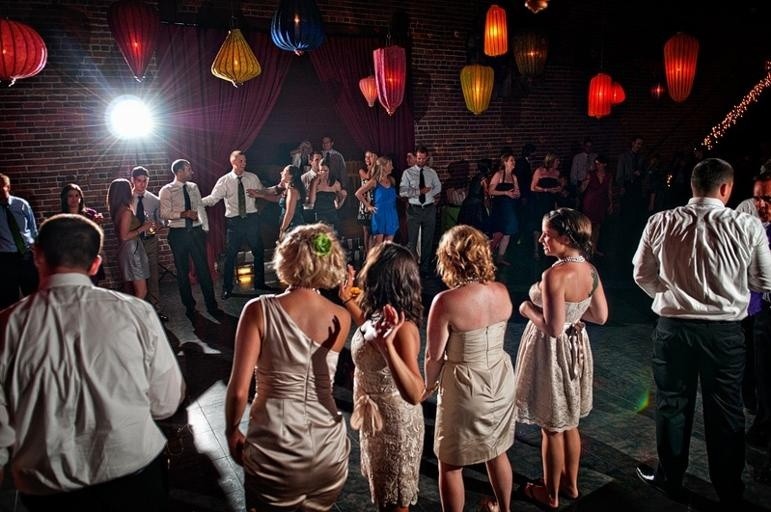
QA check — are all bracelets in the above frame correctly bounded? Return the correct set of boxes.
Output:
[135,229,141,236]
[343,296,354,304]
[425,381,439,392]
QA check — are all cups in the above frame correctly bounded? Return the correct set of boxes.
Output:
[191,207,199,221]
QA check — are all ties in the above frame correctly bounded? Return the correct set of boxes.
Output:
[134,196,146,240]
[183,184,193,230]
[326,152,330,167]
[419,168,427,205]
[237,175,247,219]
[4,206,27,259]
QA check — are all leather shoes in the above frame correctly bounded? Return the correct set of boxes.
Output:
[185,296,196,316]
[637,466,683,501]
[158,312,170,321]
[221,289,233,300]
[253,280,275,292]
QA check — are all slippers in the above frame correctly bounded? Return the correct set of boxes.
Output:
[538,475,583,502]
[519,481,560,511]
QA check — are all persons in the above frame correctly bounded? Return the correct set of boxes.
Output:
[0,214,188,511]
[0,171,38,310]
[419,223,520,512]
[158,156,219,317]
[288,136,705,281]
[631,156,771,510]
[511,205,610,512]
[60,183,107,288]
[107,175,150,299]
[127,165,168,323]
[335,238,425,512]
[245,164,306,242]
[200,150,283,300]
[224,221,356,512]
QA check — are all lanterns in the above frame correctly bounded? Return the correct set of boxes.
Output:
[1,15,50,89]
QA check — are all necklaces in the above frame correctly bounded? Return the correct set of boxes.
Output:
[552,255,587,267]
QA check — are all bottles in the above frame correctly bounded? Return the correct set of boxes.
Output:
[145,212,156,235]
